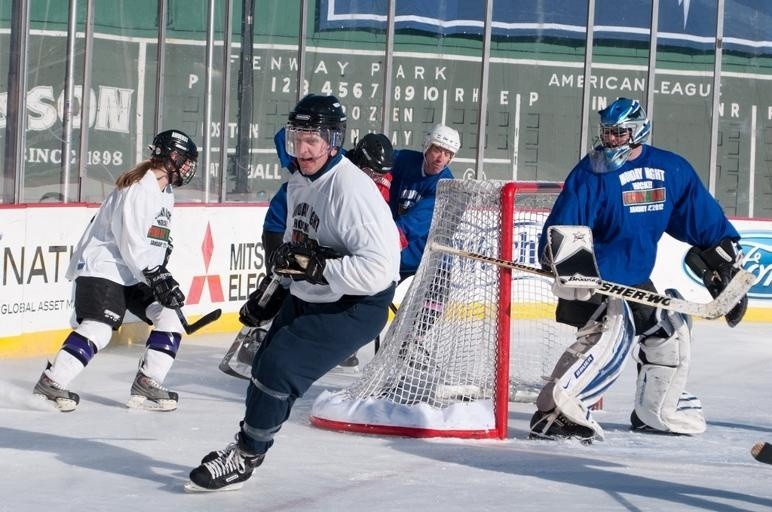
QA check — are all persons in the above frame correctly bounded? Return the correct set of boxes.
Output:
[32,129,199,412]
[184,94,401,492]
[527,97,748,442]
[392,126,469,370]
[237,128,407,367]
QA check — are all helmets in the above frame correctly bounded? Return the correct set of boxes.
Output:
[422,124,462,169]
[355,133,394,174]
[150,129,199,188]
[284,93,347,178]
[589,96,651,174]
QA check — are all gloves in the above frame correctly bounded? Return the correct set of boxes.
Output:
[238,275,292,327]
[265,237,343,285]
[542,224,602,302]
[143,265,185,309]
[682,236,748,327]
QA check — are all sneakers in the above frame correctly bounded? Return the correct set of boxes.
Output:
[527,408,596,447]
[124,369,180,403]
[29,370,81,405]
[188,434,269,490]
[629,404,708,438]
